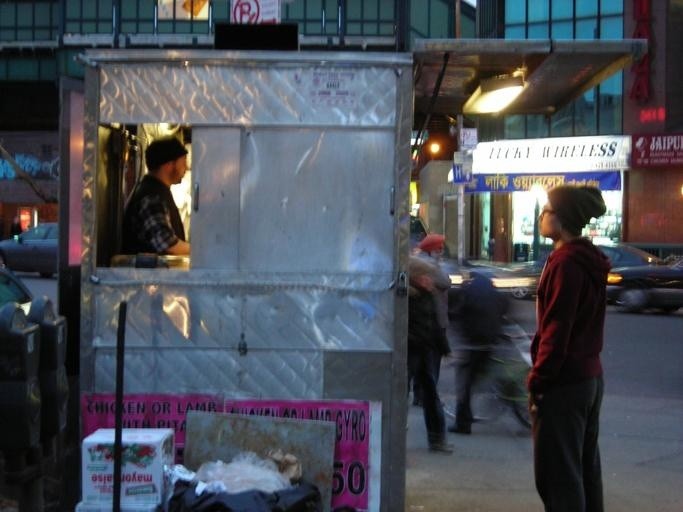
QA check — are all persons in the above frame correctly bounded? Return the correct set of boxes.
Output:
[524,182,612,511]
[10,215,21,239]
[404,232,452,409]
[445,269,517,436]
[121,135,191,255]
[405,256,454,454]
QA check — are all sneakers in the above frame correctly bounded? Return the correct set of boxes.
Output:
[448,424,472,435]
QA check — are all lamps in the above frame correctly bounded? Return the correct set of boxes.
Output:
[462,74,530,116]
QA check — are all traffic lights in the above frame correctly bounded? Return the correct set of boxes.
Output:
[423,133,456,160]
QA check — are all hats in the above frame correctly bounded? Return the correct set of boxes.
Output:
[419,234,444,254]
[549,186,605,228]
[145,138,189,172]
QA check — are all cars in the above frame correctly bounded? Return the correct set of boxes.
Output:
[1,266,42,408]
[1,220,61,279]
[407,210,683,326]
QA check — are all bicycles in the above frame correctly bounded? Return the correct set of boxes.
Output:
[434,321,533,428]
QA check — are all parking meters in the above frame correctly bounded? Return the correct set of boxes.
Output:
[27,295,70,437]
[1,302,43,452]
